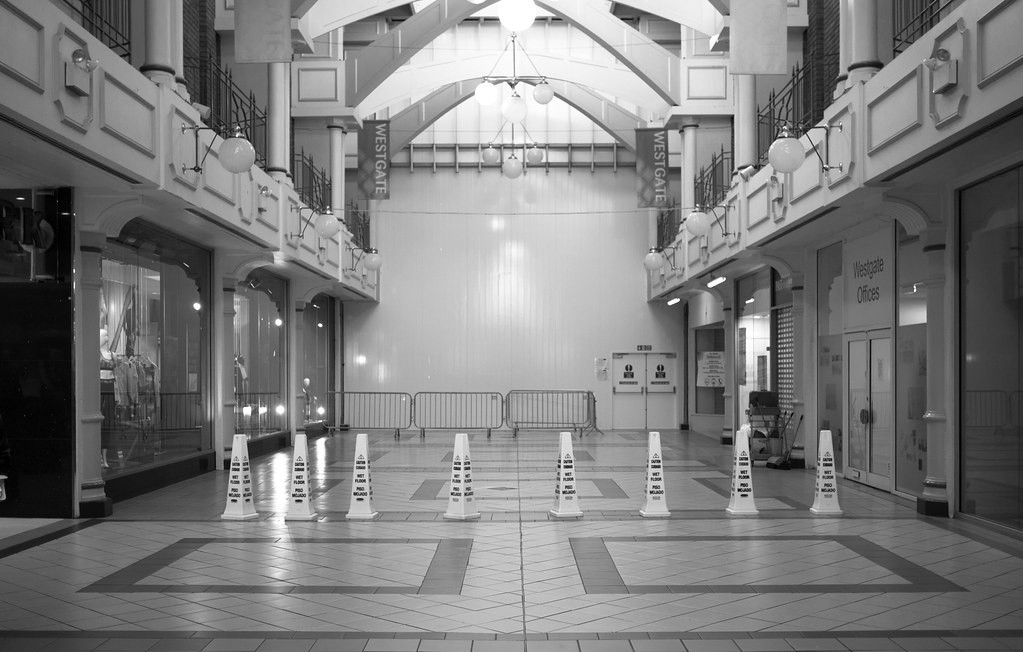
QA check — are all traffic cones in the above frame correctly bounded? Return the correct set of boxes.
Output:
[640,431,672,518]
[285,434,319,521]
[548,432,583,518]
[726,431,759,514]
[345,434,379,519]
[220,435,259,519]
[810,430,844,515]
[444,433,481,520]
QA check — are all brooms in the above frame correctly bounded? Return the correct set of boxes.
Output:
[756,399,804,470]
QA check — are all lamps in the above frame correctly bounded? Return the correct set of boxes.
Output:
[169,120,256,181]
[661,286,683,306]
[347,247,383,271]
[768,176,783,201]
[645,244,680,273]
[707,268,728,291]
[768,122,843,180]
[290,205,339,240]
[471,2,553,176]
[63,50,98,99]
[687,203,736,237]
[259,185,271,210]
[925,45,959,94]
